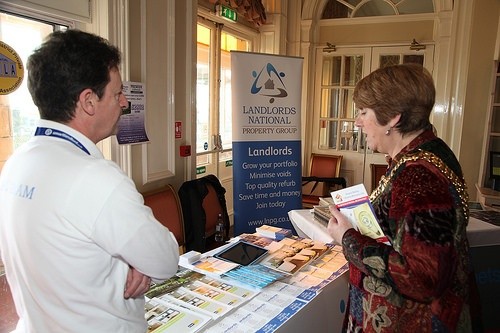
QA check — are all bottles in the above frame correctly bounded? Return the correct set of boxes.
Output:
[214,213,224,241]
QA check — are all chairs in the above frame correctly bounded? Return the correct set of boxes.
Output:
[141,183,186,254]
[179,175,230,252]
[369,163,387,192]
[302,153,343,209]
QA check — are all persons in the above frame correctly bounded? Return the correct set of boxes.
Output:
[327,62,477,333]
[0,28,179,333]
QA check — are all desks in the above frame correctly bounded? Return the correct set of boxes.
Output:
[144,235,354,333]
[466,208,500,248]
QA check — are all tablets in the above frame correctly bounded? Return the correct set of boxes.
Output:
[215,241,269,266]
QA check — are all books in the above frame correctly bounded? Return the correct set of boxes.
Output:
[143,224,349,333]
[313,198,335,227]
